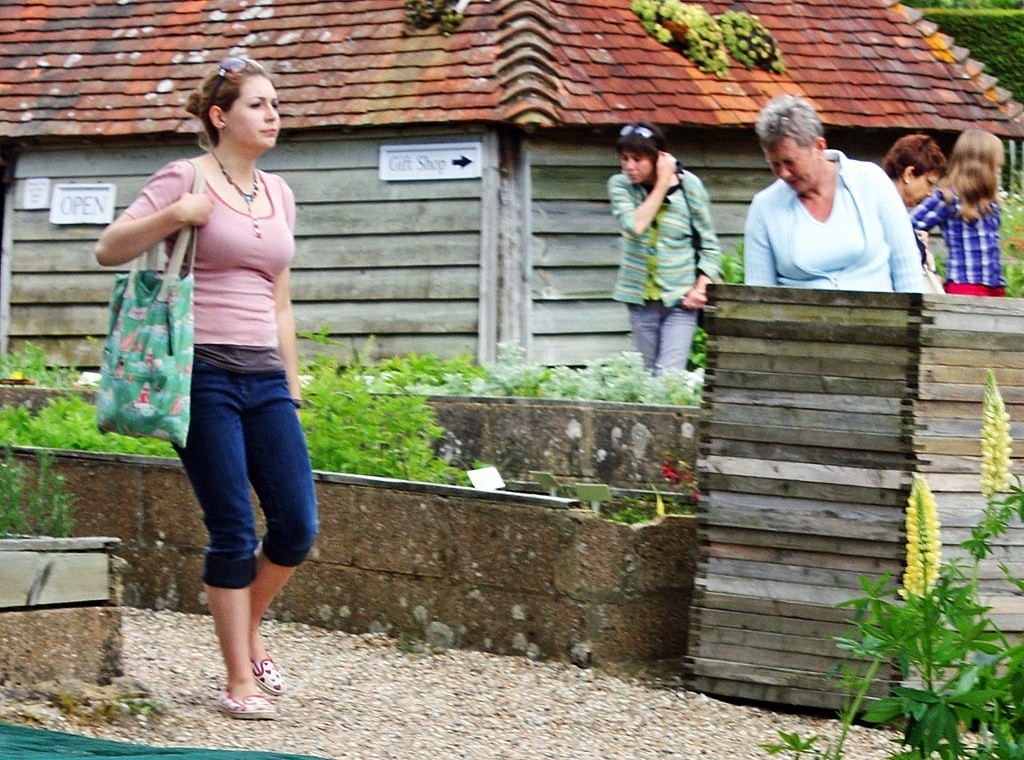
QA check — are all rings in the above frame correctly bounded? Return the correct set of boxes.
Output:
[691,303,695,306]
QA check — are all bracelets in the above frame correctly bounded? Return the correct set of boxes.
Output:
[693,284,706,295]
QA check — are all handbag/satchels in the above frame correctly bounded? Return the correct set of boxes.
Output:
[97,162,206,449]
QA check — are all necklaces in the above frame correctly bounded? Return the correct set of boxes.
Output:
[211,151,262,240]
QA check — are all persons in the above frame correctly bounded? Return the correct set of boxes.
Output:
[885,134,946,267]
[93,58,319,718]
[606,122,721,377]
[743,94,924,292]
[909,129,1008,298]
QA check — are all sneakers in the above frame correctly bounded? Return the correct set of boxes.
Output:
[216,687,276,720]
[251,651,287,696]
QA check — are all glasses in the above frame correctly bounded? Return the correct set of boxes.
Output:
[618,124,658,152]
[211,56,265,107]
